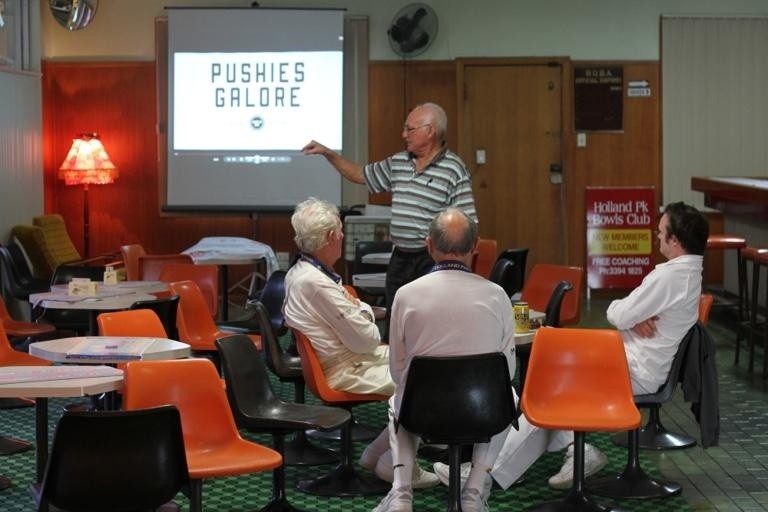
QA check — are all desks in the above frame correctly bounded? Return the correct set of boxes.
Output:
[514,327,553,395]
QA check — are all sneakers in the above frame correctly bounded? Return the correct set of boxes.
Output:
[359,444,609,511]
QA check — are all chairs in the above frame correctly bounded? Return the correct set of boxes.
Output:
[473,240,496,278]
[521,264,582,325]
[521,327,641,508]
[397,352,519,512]
[610,294,712,449]
[488,247,529,296]
[489,258,513,288]
[585,424,683,500]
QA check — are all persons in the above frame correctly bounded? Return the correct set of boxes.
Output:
[433,202,710,492]
[281,197,441,489]
[370,206,520,512]
[301,103,479,344]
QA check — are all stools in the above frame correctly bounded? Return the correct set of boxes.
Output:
[702,234,750,346]
[740,244,768,377]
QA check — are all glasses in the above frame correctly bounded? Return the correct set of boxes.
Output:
[400,124,430,133]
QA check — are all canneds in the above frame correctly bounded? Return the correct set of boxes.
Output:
[514,302,529,334]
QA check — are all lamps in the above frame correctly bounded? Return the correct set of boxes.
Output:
[59,134,115,260]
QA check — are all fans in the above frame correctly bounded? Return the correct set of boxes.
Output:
[384,3,439,58]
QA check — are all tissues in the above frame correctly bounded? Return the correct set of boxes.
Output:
[103,267,118,285]
[68,277,100,296]
